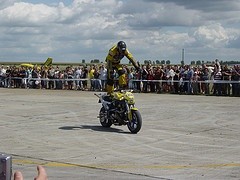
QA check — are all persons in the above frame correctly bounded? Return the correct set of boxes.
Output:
[118,63,240,97]
[105,40,140,92]
[0,64,119,92]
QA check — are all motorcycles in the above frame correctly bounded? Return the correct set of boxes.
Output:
[94,88,142,134]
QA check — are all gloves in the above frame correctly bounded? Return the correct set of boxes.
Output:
[110,71,116,81]
[136,67,143,75]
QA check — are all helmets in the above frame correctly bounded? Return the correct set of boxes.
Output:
[118,41,126,50]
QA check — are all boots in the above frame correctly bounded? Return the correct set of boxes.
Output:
[119,74,126,85]
[107,84,114,98]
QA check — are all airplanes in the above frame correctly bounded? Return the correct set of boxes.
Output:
[20,57,53,71]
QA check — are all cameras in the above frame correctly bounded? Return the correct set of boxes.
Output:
[0,154,12,180]
